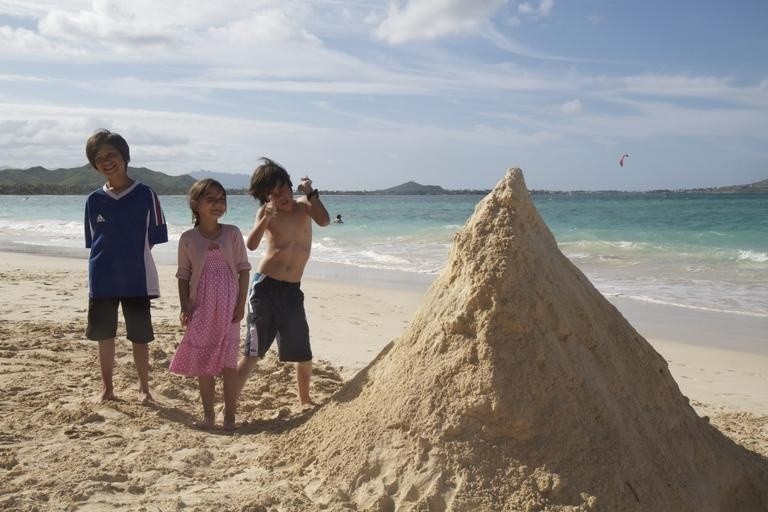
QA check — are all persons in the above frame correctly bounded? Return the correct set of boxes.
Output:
[85,129,167,406]
[235,157,330,406]
[169,178,251,431]
[334,214,343,223]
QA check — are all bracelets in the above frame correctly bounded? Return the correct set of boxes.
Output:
[308,188,319,201]
